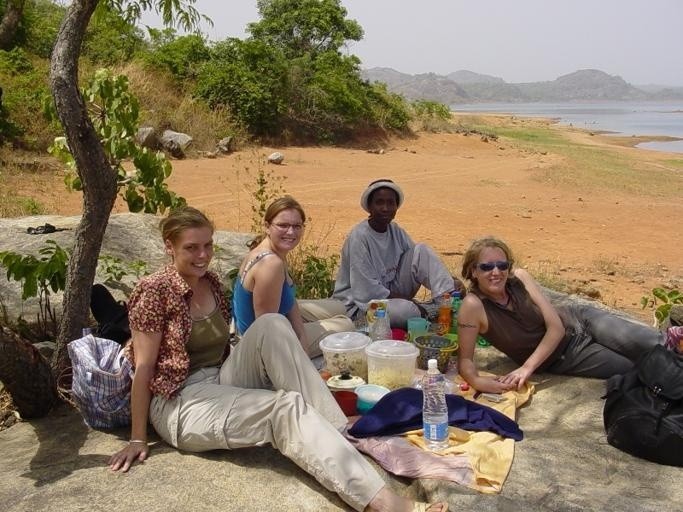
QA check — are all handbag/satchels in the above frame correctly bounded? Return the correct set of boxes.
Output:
[66,334,134,429]
[599,343,683,467]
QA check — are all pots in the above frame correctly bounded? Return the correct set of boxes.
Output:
[326,370,366,391]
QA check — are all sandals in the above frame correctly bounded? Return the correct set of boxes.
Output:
[407,500,449,512]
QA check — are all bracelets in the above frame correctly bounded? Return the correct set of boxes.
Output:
[129,439,147,443]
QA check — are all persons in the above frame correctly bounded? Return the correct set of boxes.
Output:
[108,206,451,512]
[330,178,466,331]
[457,236,673,393]
[232,194,354,360]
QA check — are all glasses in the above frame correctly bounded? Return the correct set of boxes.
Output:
[271,222,303,231]
[474,260,510,271]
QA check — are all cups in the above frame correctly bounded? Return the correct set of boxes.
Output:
[335,391,357,416]
[407,317,431,341]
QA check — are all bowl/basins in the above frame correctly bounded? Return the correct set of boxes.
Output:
[354,384,391,416]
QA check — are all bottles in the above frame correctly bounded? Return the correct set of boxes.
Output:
[422,358,449,452]
[414,373,470,394]
[437,291,451,335]
[452,291,461,334]
[366,302,392,340]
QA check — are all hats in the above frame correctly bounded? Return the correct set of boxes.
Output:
[359,178,404,214]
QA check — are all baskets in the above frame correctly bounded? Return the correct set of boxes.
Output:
[413,335,459,374]
